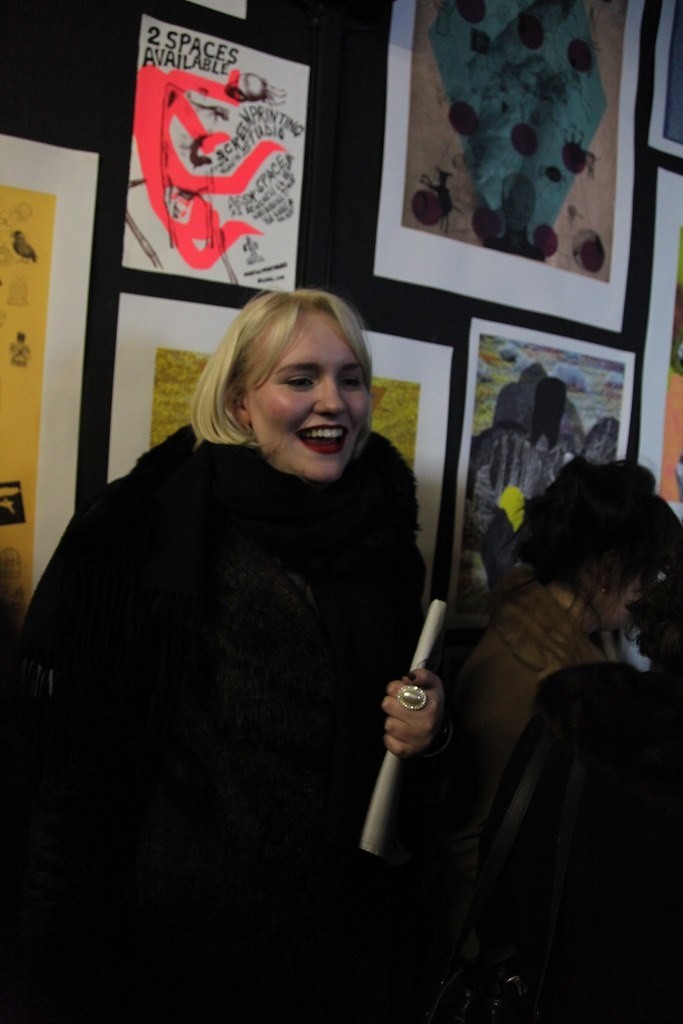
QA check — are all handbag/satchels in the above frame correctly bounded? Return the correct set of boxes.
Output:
[425,718,588,1023]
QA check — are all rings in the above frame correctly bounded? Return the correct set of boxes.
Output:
[397,684,429,710]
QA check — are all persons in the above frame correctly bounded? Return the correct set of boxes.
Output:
[440,451,683,1024]
[2,285,482,1024]
